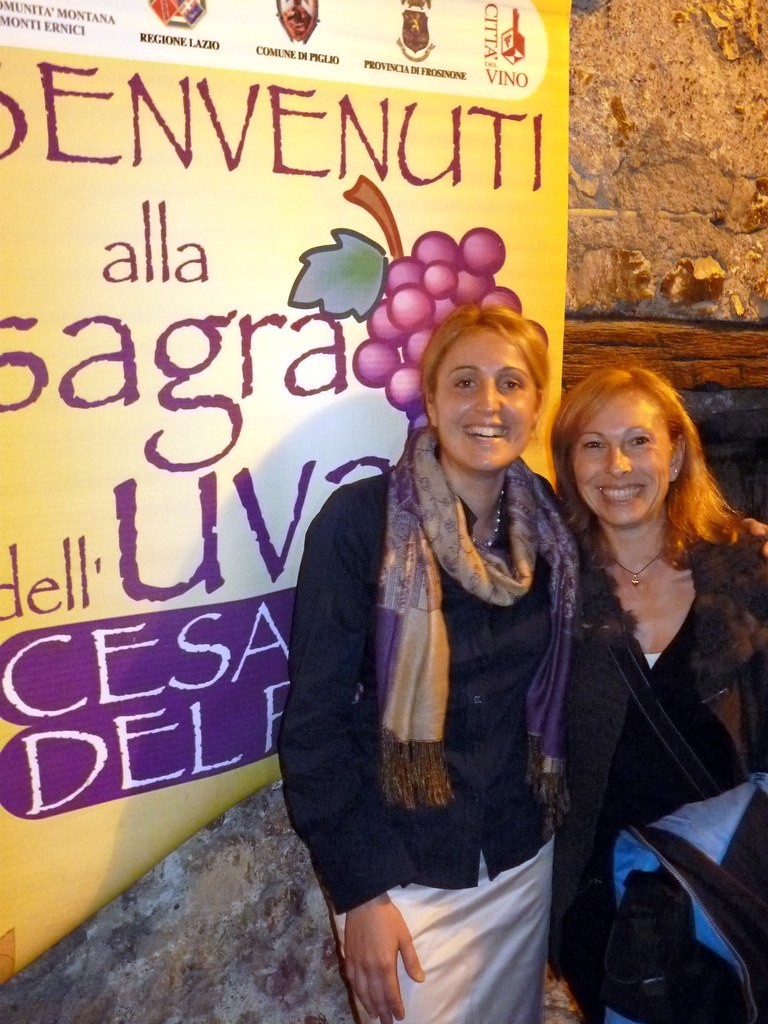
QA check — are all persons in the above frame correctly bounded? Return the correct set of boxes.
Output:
[277,303,581,1024]
[551,366,768,1024]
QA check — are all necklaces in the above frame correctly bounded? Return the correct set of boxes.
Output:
[610,552,662,586]
[471,491,504,547]
